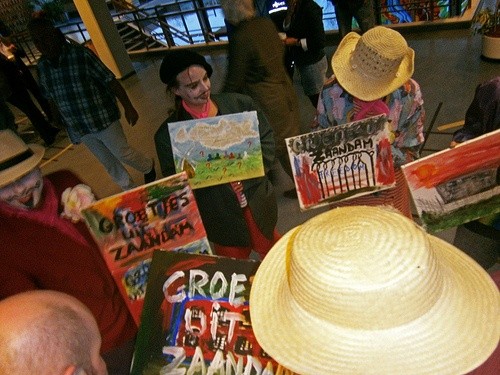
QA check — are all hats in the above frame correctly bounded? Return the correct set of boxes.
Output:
[249,205,500,375]
[159,48,213,84]
[0,129,45,188]
[331,26,415,101]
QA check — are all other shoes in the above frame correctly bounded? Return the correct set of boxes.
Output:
[144,158,156,184]
[311,112,319,128]
[283,188,298,199]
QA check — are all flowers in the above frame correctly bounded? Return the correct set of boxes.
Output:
[470,0,500,40]
[58,183,96,223]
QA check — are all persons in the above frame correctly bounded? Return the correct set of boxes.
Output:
[281,0,328,110]
[31,18,157,191]
[0,128,138,375]
[221,0,299,200]
[0,27,64,145]
[310,24,425,219]
[0,287,111,375]
[153,47,283,259]
[266,0,384,87]
[448,74,500,277]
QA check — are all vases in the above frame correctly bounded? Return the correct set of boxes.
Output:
[481,33,500,59]
